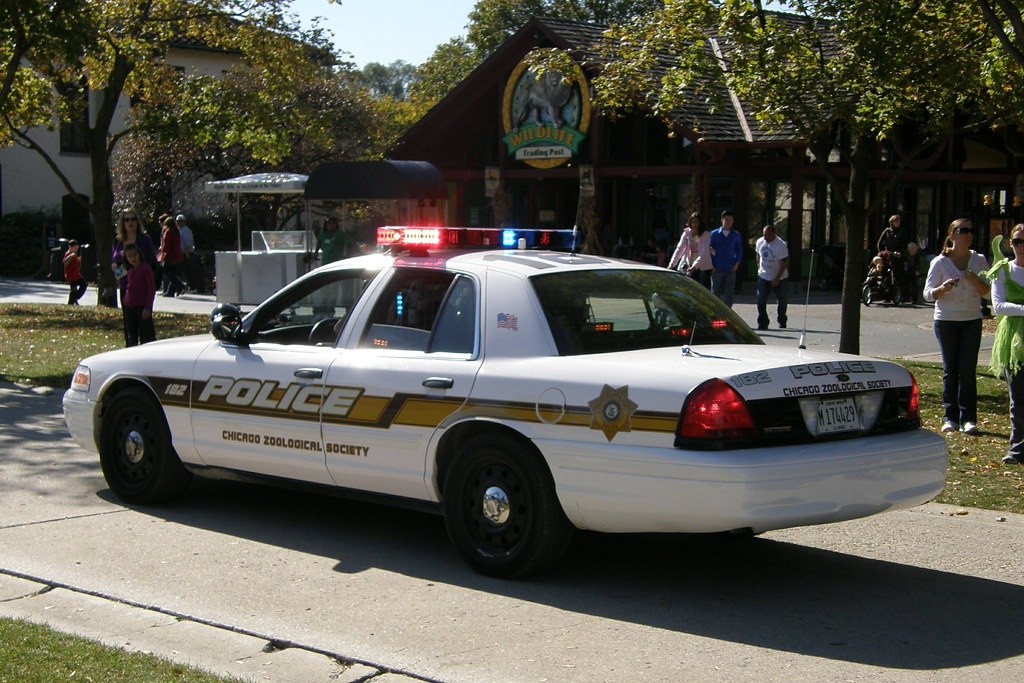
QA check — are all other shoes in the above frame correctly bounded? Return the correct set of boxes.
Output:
[163,292,174,297]
[778,324,787,328]
[757,323,768,330]
[176,282,187,296]
[1002,454,1018,464]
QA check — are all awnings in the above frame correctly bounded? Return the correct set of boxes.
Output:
[303,158,447,251]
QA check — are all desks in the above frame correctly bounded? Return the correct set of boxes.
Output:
[213,249,320,306]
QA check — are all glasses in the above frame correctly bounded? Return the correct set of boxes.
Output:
[1011,237,1024,247]
[954,227,975,235]
[123,216,138,222]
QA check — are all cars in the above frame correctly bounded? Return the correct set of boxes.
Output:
[60,223,950,582]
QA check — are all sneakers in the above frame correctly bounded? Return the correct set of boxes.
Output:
[941,420,960,433]
[958,421,978,433]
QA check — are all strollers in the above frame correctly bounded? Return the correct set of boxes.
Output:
[861,250,904,306]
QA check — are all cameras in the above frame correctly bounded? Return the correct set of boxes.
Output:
[949,277,960,287]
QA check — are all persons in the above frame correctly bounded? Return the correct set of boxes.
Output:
[63,239,88,305]
[666,211,716,293]
[1011,195,1021,206]
[754,224,788,329]
[990,222,1024,464]
[877,214,908,274]
[156,214,187,297]
[898,242,922,306]
[868,255,900,305]
[709,208,743,310]
[983,194,992,206]
[922,218,993,432]
[111,208,160,305]
[122,243,157,347]
[175,213,199,294]
[314,215,356,318]
[641,235,661,267]
